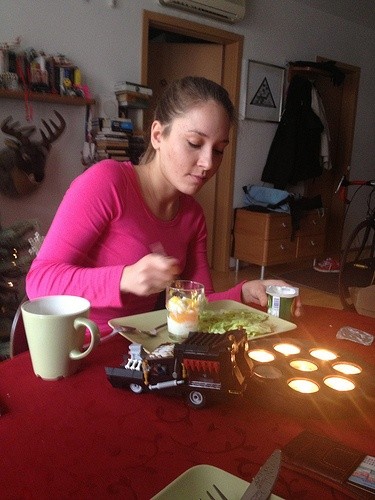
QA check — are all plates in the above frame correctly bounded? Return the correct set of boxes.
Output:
[109,299,296,356]
[149,464,285,500]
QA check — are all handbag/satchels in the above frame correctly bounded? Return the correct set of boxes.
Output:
[241,185,293,211]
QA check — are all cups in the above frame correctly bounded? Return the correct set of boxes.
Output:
[20,295,99,381]
[264,286,298,323]
[165,280,204,342]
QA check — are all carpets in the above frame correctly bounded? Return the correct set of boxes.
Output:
[275,257,375,299]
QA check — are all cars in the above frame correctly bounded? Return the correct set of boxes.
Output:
[104,324,256,410]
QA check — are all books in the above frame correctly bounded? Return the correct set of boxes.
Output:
[92,118,144,161]
[115,81,153,107]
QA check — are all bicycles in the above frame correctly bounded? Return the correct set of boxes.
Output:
[333,175,375,314]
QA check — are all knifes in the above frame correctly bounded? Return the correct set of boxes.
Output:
[239,449,282,500]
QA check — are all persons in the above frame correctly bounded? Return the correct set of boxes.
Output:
[27,77,301,349]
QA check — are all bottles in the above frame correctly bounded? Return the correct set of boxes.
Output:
[0,37,84,97]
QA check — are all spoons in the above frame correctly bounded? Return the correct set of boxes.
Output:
[139,321,167,337]
[83,325,136,350]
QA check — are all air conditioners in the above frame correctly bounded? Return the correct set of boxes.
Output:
[156,0,249,23]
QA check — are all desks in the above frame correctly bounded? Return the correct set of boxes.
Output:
[0,304,374,500]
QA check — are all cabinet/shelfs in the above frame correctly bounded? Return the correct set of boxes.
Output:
[234,209,330,279]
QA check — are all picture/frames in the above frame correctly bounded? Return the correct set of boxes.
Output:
[244,60,285,123]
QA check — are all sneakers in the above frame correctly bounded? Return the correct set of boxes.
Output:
[313,258,339,272]
